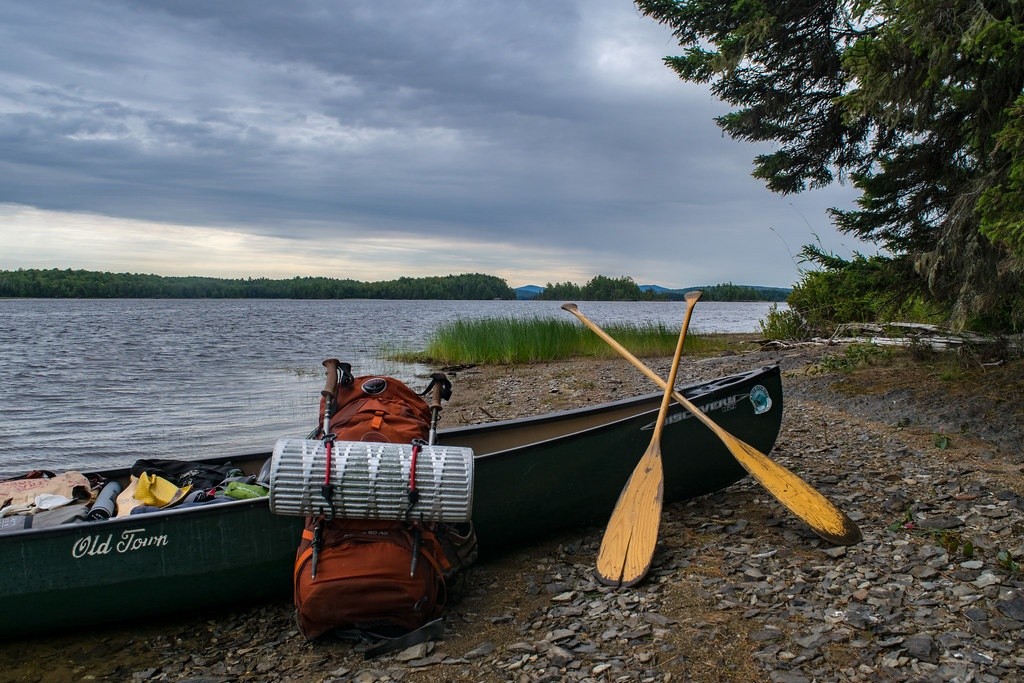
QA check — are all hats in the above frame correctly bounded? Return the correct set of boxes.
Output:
[133,470,194,510]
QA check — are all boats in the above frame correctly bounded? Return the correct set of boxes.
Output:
[0,360,788,649]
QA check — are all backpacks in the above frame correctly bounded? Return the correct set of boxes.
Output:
[130,456,245,491]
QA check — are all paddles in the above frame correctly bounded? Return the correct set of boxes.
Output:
[560,291,864,589]
